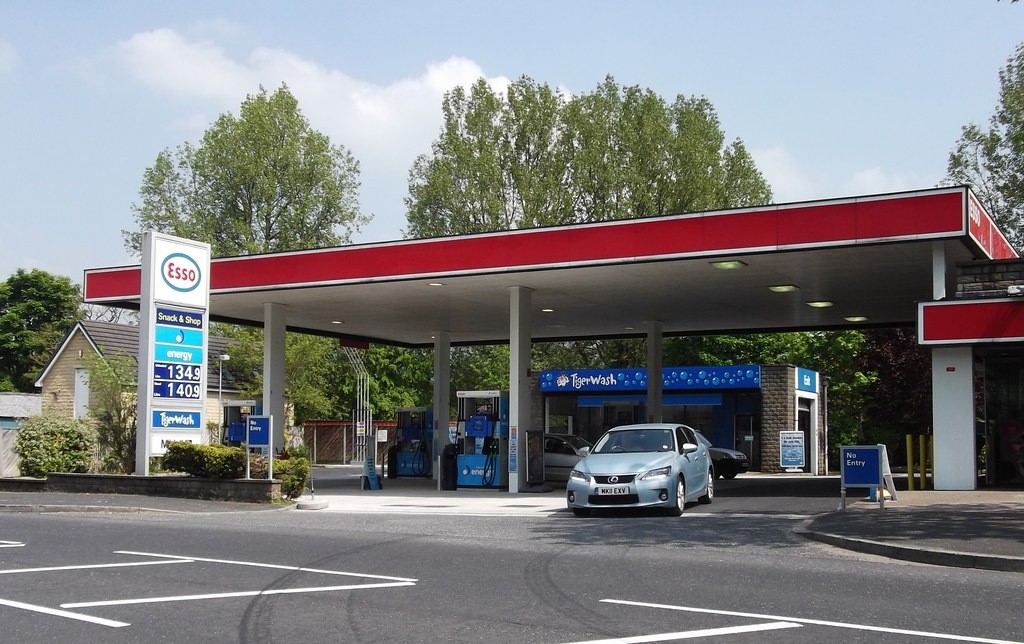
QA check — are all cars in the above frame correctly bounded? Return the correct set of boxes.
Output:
[565,424,716,516]
[694,429,747,480]
[540,433,598,481]
